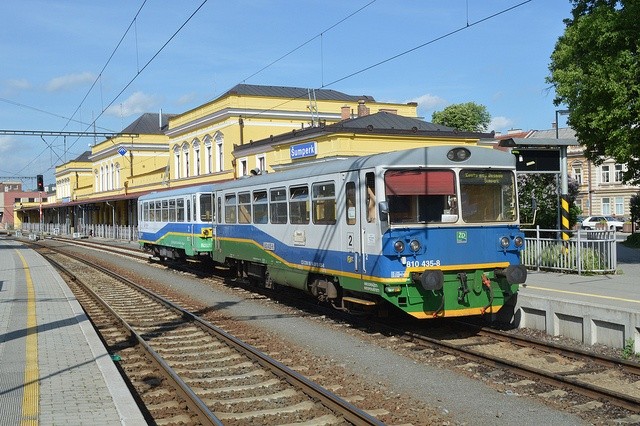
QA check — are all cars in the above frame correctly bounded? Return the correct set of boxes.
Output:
[581,216,624,232]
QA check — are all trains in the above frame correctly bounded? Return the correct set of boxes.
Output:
[137,145,527,328]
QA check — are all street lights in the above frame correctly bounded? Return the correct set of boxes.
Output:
[555,110,572,245]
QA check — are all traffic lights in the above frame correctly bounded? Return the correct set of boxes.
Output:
[37,175,44,191]
